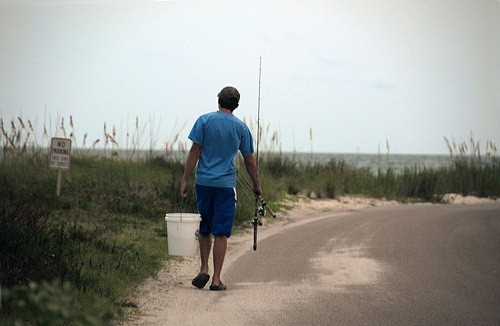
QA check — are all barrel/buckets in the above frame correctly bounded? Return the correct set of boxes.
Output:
[165,188,201,257]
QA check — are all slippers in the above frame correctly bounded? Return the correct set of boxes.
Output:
[192,272,210,289]
[210,280,227,290]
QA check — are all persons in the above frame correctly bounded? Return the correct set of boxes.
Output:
[180,86,262,291]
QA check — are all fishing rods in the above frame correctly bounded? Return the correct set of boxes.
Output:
[252,57,264,250]
[235,164,278,219]
